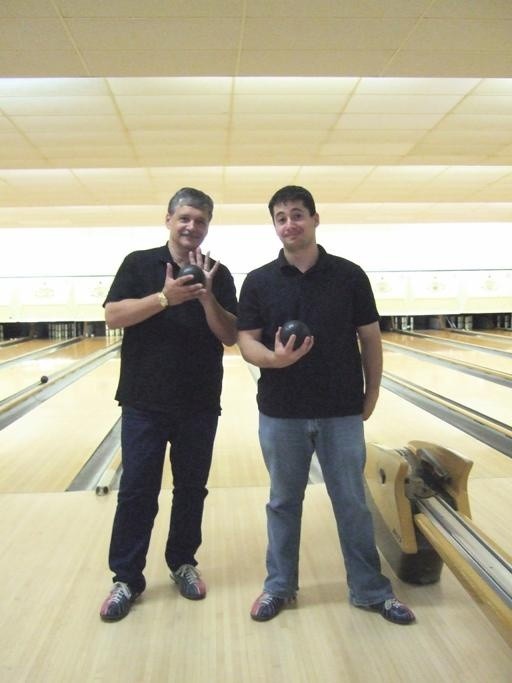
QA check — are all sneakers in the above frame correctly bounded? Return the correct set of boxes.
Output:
[352,591,415,624]
[251,587,297,622]
[169,560,207,600]
[99,577,145,622]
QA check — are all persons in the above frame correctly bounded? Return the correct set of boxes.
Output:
[234,181,417,625]
[96,186,239,622]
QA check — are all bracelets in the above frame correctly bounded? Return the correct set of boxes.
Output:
[158,289,172,310]
